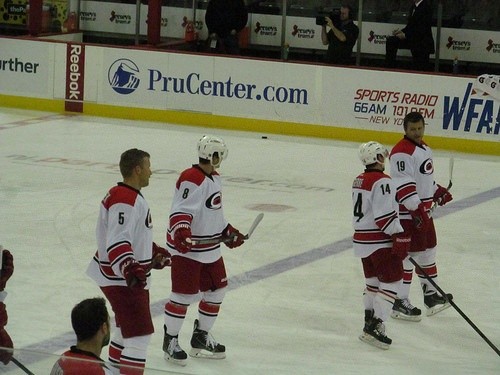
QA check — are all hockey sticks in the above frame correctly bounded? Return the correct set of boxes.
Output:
[9,356,35,375]
[408,256,500,356]
[412,157,453,227]
[128,254,162,287]
[191,213,264,246]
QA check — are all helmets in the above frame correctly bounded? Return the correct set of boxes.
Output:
[358,140,389,166]
[196,136,228,168]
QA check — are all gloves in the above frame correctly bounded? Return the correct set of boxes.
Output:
[151,242,173,270]
[0,328,14,365]
[0,249,14,292]
[120,258,147,289]
[173,224,194,253]
[0,302,9,329]
[407,203,431,233]
[433,184,453,206]
[222,224,247,249]
[391,231,411,260]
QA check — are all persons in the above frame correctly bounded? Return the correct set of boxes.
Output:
[0,245,14,365]
[320,4,359,64]
[203,0,248,55]
[351,140,410,350]
[389,112,455,321]
[162,133,245,366]
[50,296,114,375]
[386,0,435,72]
[87,148,172,375]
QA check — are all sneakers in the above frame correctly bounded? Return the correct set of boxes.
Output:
[161,323,187,367]
[188,319,227,359]
[359,310,392,350]
[391,299,422,321]
[421,282,453,318]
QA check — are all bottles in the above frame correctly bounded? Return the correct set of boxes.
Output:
[453,57,458,73]
[283,43,289,60]
